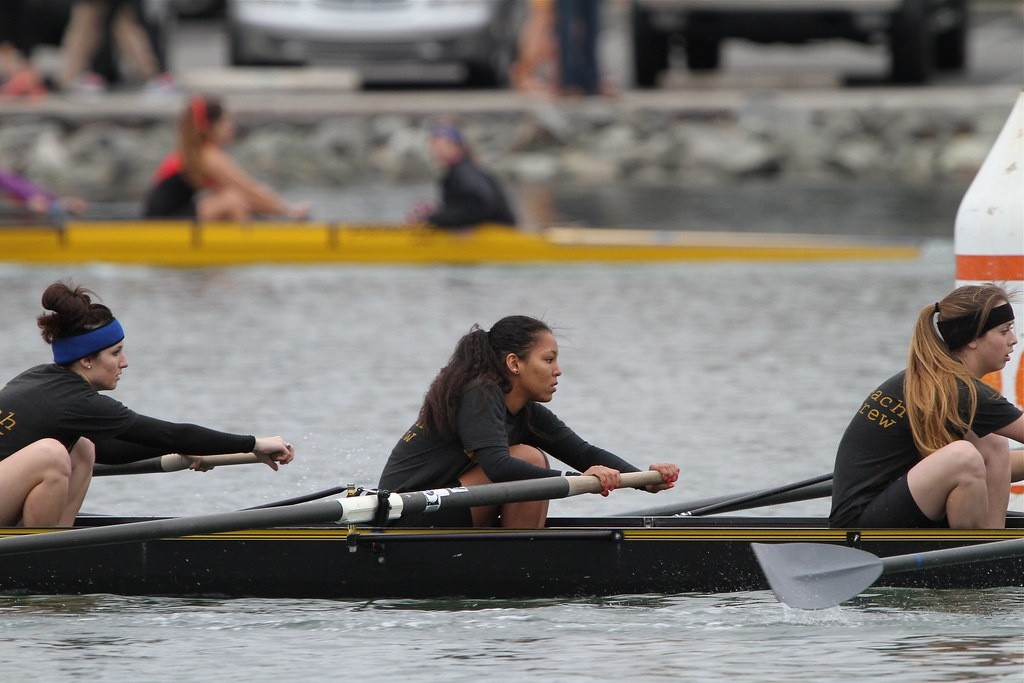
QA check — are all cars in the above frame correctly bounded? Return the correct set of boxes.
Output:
[605,1,977,89]
[215,1,532,94]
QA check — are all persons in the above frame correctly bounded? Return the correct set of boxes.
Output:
[415,120,515,233]
[140,97,311,220]
[0,0,175,98]
[0,284,294,527]
[0,169,85,214]
[828,284,1024,530]
[550,0,613,103]
[378,315,681,528]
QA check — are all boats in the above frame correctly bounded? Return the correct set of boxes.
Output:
[0,508,1024,605]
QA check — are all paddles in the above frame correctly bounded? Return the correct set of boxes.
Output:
[751,538,1024,610]
[0,470,664,556]
[93,449,283,477]
[610,478,834,518]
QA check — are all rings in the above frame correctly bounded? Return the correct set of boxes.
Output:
[286,444,291,449]
